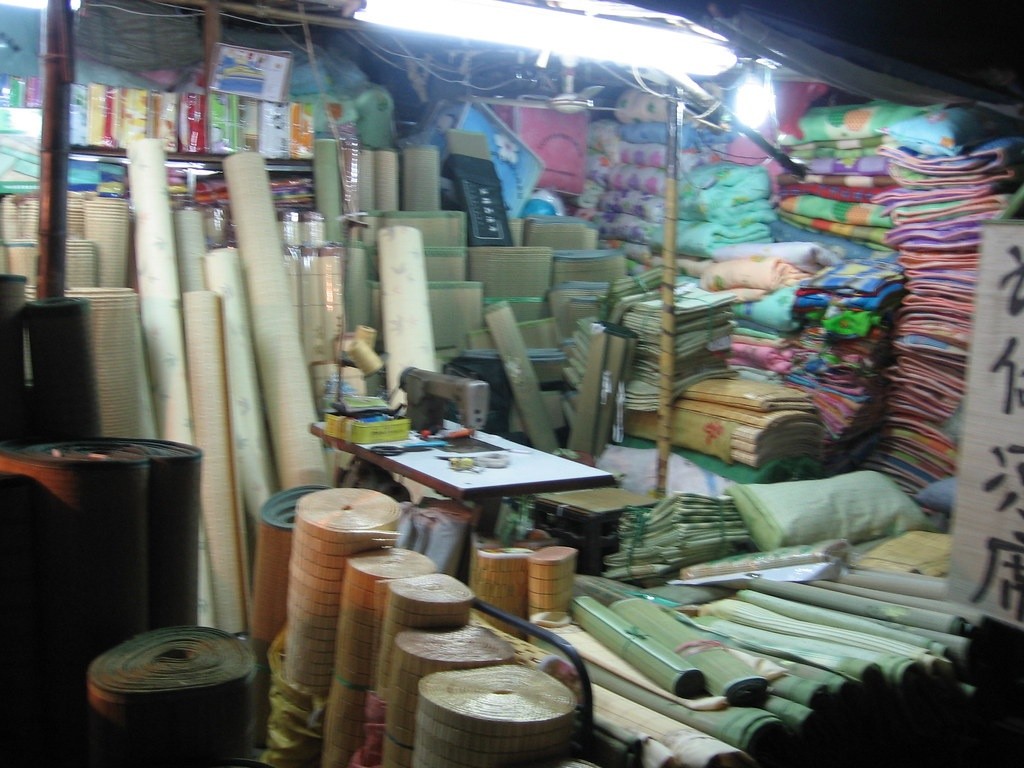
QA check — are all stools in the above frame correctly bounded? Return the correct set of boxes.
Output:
[528,486,658,580]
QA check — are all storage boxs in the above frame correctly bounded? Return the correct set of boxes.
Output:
[425,96,589,212]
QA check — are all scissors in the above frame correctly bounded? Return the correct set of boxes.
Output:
[371,444,431,457]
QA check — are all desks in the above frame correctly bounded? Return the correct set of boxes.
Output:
[309,410,615,581]
